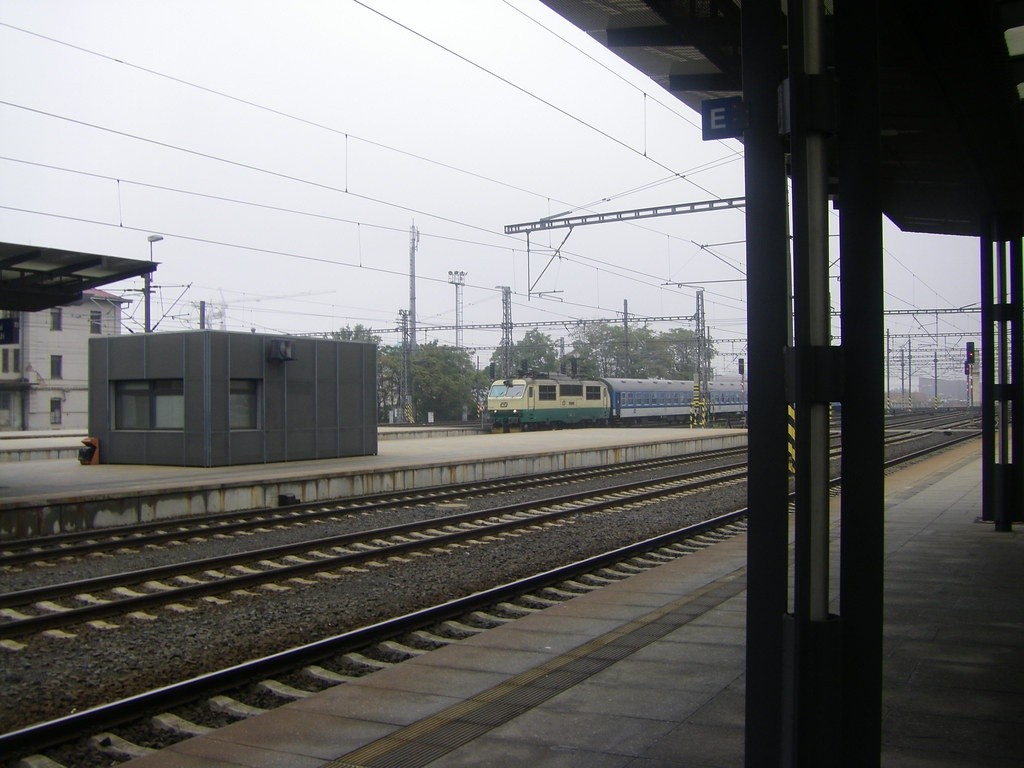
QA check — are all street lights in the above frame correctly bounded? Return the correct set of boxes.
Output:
[140,236,167,333]
[448,270,465,348]
[396,308,414,422]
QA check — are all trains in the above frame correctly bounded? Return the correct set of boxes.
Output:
[486,374,749,427]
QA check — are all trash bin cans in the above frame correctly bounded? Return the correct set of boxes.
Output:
[79,437,99,465]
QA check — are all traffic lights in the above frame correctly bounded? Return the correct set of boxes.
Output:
[738,359,744,374]
[968,342,974,363]
[965,361,970,375]
